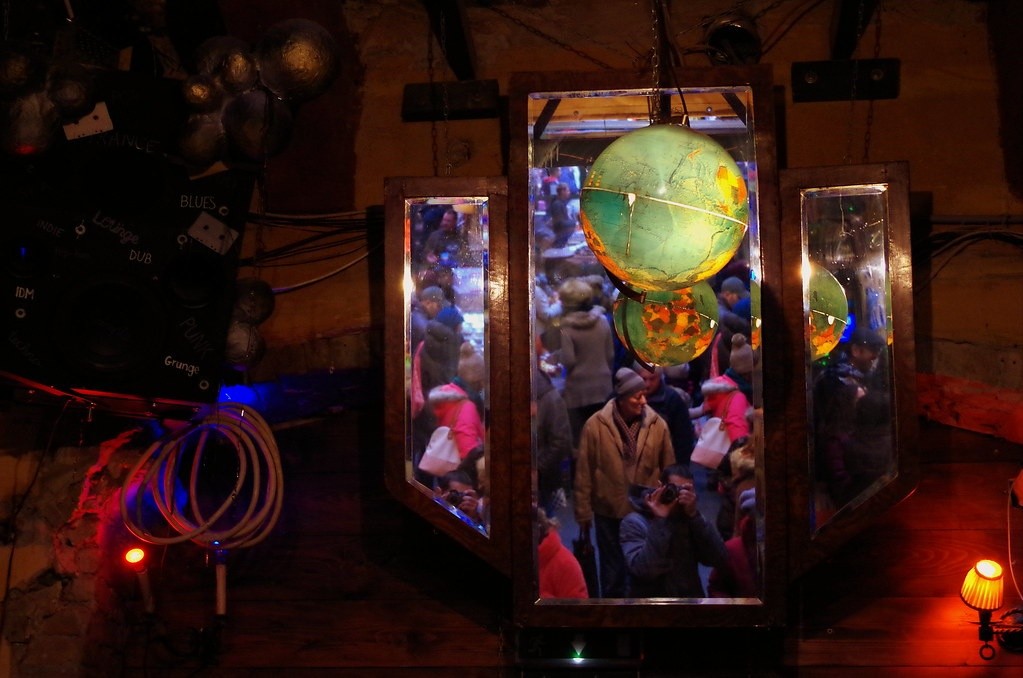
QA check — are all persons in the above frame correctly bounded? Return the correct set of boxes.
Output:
[404,134,899,599]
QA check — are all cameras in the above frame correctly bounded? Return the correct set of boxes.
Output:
[661,483,680,503]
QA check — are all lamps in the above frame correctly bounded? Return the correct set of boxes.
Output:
[960,559,1005,628]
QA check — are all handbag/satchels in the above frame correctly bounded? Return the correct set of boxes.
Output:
[418,397,471,477]
[572,524,600,597]
[716,473,754,539]
[539,458,576,496]
[690,388,741,468]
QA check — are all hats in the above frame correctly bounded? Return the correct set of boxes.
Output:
[613,367,648,400]
[721,275,751,298]
[851,325,886,351]
[457,341,486,383]
[420,286,451,308]
[732,297,751,321]
[730,332,753,373]
[436,306,463,332]
[814,377,858,414]
